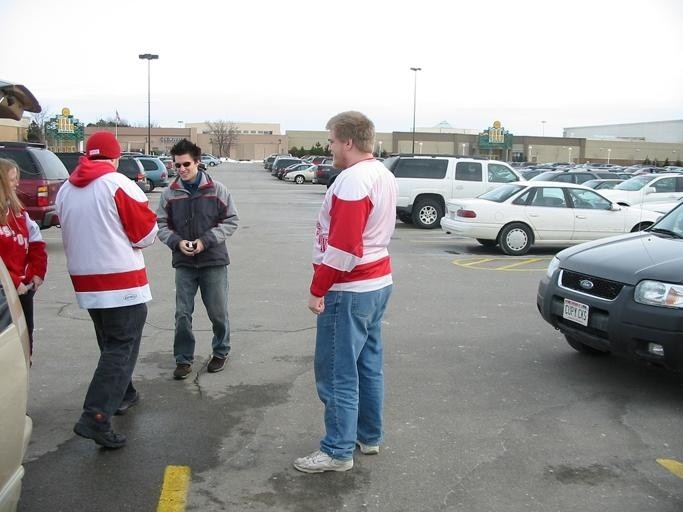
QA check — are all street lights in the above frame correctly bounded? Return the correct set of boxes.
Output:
[409,67,422,154]
[138,53,159,155]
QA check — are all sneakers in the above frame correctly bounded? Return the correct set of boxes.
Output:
[207,353,228,372]
[73,415,126,448]
[115,391,139,414]
[355,439,379,455]
[292,450,353,474]
[173,364,192,379]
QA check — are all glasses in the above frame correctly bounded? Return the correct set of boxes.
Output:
[174,162,190,168]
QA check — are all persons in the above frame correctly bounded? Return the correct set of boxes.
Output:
[0,158,48,368]
[294,111,400,473]
[582,160,591,168]
[157,138,240,378]
[54,132,161,449]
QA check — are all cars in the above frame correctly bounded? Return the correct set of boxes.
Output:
[537,200,683,370]
[263,151,342,189]
[440,179,667,257]
[72,148,225,192]
[510,162,683,203]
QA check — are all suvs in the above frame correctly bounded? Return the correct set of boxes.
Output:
[0,142,71,228]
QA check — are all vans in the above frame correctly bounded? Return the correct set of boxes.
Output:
[383,157,523,229]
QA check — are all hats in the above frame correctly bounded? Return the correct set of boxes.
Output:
[86,131,120,158]
